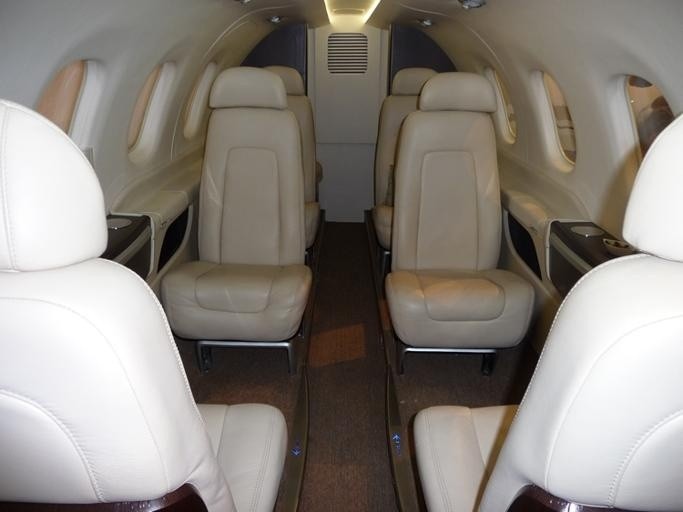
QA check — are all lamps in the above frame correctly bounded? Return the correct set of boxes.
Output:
[323,0,381,33]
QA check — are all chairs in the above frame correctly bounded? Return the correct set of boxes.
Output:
[262,65,320,253]
[0,99,290,512]
[160,66,312,379]
[372,66,435,280]
[384,72,535,376]
[412,113,683,512]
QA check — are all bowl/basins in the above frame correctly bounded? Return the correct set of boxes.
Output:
[602,237,635,257]
